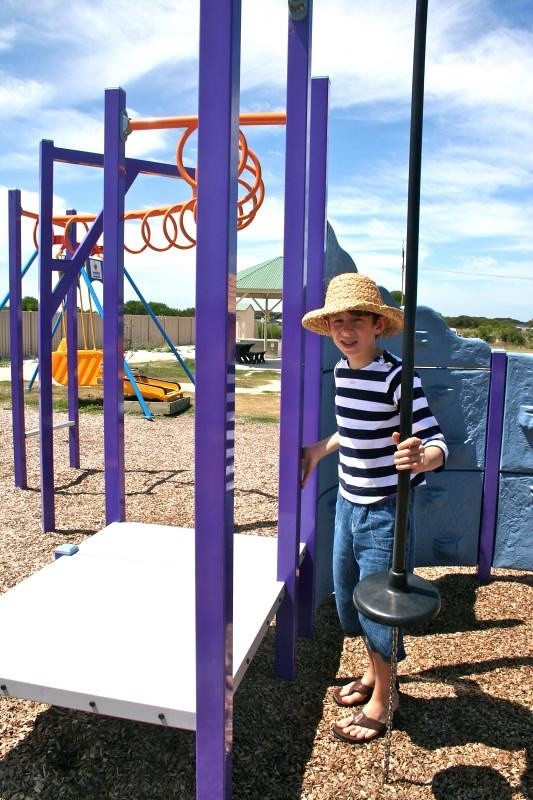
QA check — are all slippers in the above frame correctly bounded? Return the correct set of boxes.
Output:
[332,710,401,744]
[333,679,399,707]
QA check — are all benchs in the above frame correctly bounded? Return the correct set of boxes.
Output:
[245,351,266,363]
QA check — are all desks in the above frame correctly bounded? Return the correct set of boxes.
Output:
[236,342,256,363]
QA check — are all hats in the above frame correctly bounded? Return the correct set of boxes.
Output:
[301,272,404,339]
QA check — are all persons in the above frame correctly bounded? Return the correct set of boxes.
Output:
[300,273,448,742]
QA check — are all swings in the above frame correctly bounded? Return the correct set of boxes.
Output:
[50,254,103,386]
